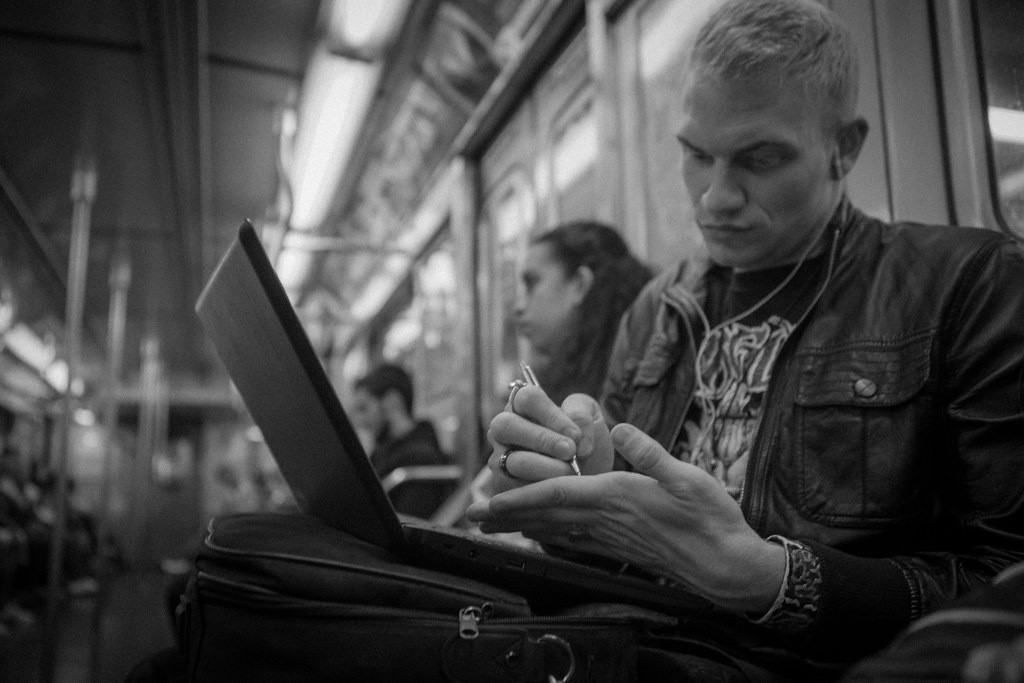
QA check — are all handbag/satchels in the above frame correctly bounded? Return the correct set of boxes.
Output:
[164,505,770,682]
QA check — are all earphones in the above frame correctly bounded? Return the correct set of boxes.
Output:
[834,144,841,166]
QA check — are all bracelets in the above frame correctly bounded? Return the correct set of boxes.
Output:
[743,534,820,636]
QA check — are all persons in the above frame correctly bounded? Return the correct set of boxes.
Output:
[468,217,662,557]
[0,404,296,643]
[468,1,1023,674]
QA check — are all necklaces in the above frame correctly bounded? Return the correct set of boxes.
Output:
[352,365,462,524]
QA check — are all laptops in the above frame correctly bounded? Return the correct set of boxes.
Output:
[195,218,718,615]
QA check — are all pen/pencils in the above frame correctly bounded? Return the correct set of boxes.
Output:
[518,359,584,477]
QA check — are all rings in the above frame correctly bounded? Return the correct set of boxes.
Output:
[507,379,530,418]
[499,447,525,481]
